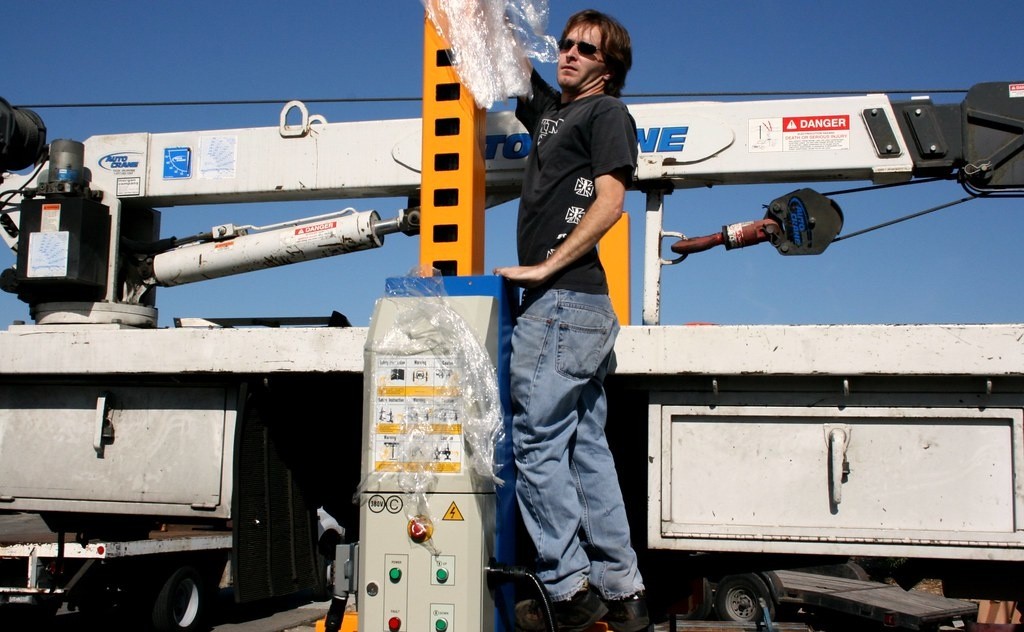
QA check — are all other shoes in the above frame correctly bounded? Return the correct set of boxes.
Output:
[589,583,650,632]
[514,589,608,632]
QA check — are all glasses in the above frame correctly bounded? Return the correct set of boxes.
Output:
[558,39,600,55]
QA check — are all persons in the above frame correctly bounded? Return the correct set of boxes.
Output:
[476,0,650,632]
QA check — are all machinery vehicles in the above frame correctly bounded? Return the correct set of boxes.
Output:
[0,81,1023,632]
